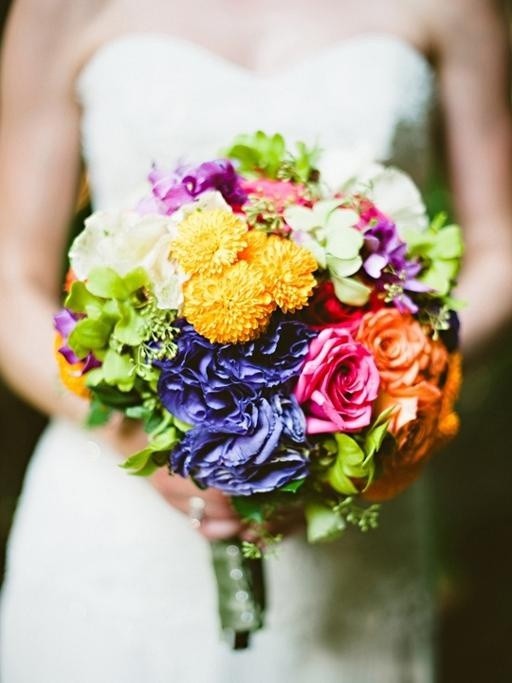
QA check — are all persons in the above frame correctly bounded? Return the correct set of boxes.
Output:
[1,1,512,683]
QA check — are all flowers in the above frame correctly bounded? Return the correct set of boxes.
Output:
[50,132,464,649]
